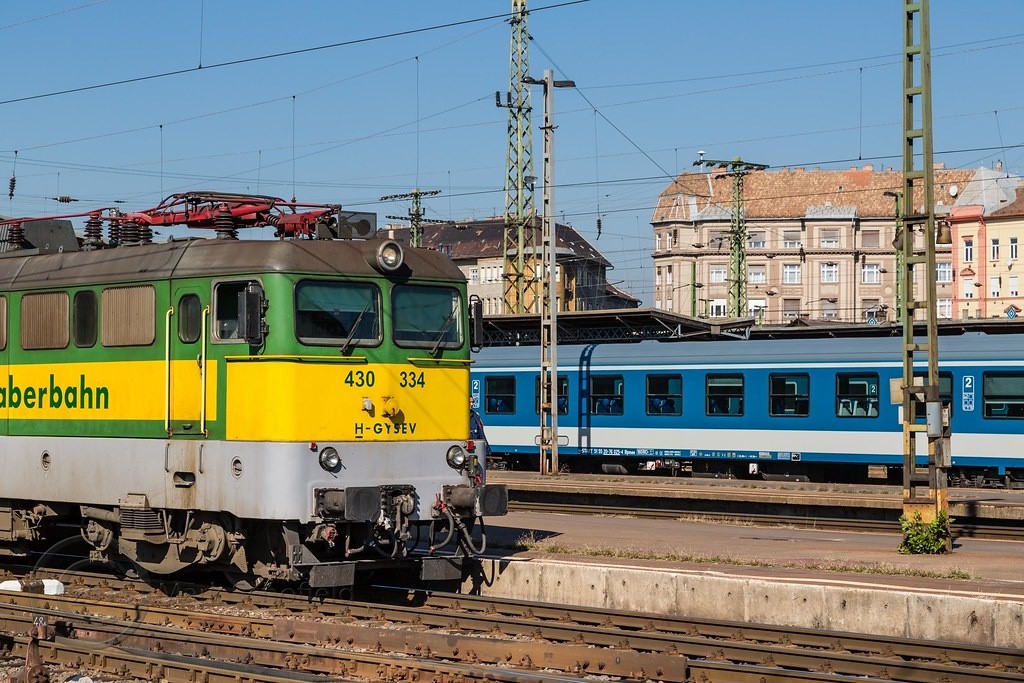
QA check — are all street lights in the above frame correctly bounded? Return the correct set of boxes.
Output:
[881,190,905,324]
[518,67,580,480]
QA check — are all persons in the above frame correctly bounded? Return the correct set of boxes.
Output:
[469,396,493,455]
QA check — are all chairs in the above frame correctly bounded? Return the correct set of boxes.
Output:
[488,394,808,417]
[836,398,879,417]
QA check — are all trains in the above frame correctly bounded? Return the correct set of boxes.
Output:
[0,189,508,591]
[468,327,1024,491]
